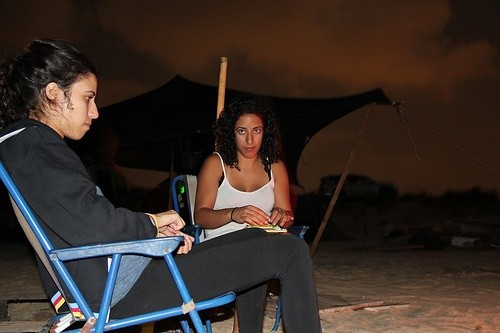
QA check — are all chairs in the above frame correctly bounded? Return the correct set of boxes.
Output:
[0,164,236,333]
[172,176,309,333]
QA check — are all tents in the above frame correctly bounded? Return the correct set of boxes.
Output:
[81,75,405,192]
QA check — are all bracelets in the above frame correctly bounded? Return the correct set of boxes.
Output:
[231,207,238,222]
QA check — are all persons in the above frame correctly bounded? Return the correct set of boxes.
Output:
[0,38,323,333]
[194,98,294,333]
